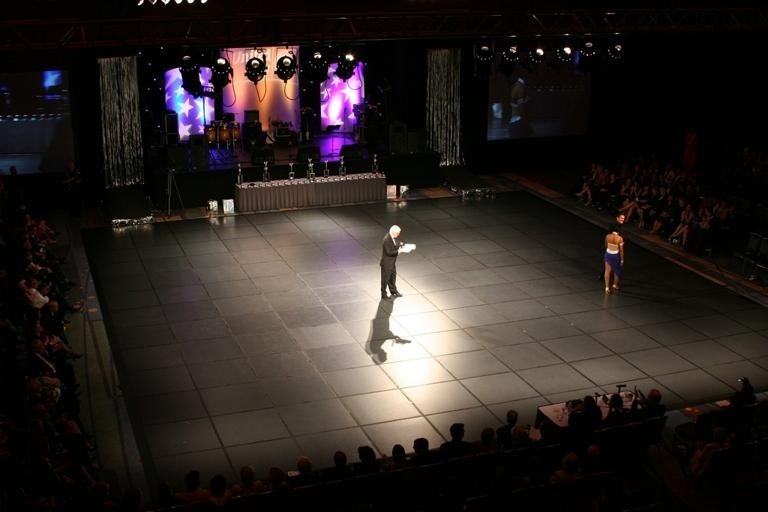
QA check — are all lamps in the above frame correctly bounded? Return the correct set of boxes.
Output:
[178,45,359,99]
[473,36,625,65]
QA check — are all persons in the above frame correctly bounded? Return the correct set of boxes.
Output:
[510,70,532,124]
[121,375,767,510]
[379,224,407,299]
[1,165,104,512]
[601,223,625,296]
[573,146,739,254]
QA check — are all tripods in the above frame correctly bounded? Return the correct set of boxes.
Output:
[166,145,187,217]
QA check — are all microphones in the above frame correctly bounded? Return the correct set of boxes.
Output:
[399,241,404,249]
[616,384,627,388]
[595,391,600,397]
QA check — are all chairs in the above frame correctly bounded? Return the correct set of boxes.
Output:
[585,414,670,444]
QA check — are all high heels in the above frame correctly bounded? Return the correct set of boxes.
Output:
[605,284,621,295]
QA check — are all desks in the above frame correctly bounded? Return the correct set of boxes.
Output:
[536,390,647,439]
[234,172,386,212]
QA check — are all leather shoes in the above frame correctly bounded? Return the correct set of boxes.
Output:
[381,293,403,300]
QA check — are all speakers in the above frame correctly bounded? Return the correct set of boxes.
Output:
[190,134,209,177]
[251,144,363,165]
[164,113,179,145]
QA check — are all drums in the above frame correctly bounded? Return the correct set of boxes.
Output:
[219,126,231,145]
[204,125,216,144]
[230,121,241,143]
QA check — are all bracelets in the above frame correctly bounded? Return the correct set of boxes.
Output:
[620,261,624,263]
